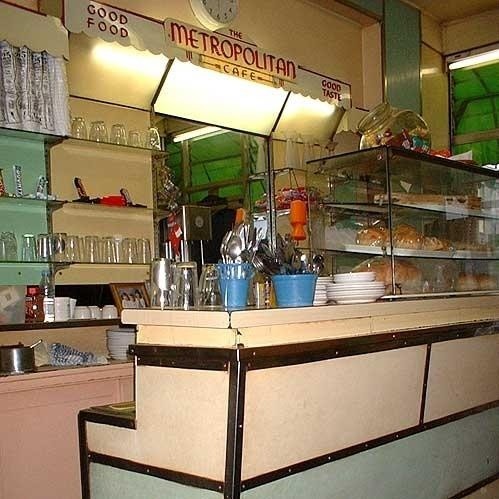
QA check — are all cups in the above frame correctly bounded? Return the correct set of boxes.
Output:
[73,304,118,321]
[254,278,275,309]
[0,40,71,137]
[149,257,225,311]
[0,229,151,265]
[217,263,251,309]
[72,116,161,150]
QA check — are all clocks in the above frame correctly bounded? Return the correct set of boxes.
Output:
[189,0,238,30]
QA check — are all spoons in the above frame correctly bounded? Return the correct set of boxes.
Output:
[219,229,325,275]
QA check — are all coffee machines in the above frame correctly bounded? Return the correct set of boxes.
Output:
[156,203,213,275]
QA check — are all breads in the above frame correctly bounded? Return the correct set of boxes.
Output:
[352,224,497,295]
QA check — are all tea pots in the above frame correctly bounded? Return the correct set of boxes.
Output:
[280,128,337,172]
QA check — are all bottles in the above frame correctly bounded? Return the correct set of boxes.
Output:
[355,102,432,156]
[24,286,44,324]
[39,270,55,324]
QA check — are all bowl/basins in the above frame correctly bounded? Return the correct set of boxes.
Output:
[272,272,317,307]
[0,344,35,371]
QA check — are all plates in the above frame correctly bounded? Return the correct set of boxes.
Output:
[110,401,136,413]
[104,326,137,361]
[310,270,386,306]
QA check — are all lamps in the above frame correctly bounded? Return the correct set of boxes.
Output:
[169,127,231,145]
[445,42,499,73]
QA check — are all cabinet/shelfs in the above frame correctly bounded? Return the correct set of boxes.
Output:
[273,167,353,254]
[307,144,496,297]
[0,126,169,333]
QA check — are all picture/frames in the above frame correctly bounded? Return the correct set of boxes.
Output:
[110,283,150,315]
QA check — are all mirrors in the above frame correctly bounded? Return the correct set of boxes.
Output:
[150,112,273,285]
